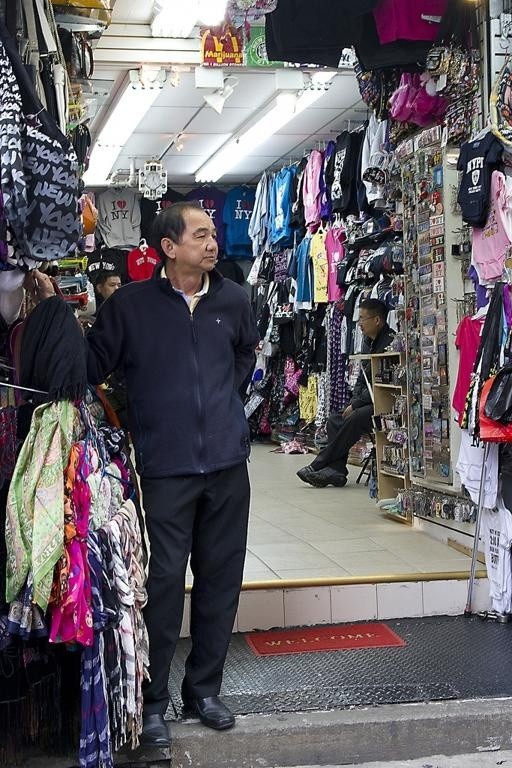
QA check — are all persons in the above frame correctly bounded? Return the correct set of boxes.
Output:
[95,269,121,299]
[296,297,398,488]
[32,200,257,749]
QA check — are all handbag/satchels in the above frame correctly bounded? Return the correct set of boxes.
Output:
[462,363,485,448]
[0,26,98,270]
[389,47,481,126]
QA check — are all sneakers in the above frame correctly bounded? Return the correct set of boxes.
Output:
[297,465,347,487]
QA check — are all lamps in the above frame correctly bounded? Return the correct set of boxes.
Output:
[192,82,330,182]
[149,0,198,39]
[81,70,165,186]
[201,76,241,116]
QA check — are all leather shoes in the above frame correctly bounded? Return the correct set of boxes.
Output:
[138,713,171,747]
[183,695,235,729]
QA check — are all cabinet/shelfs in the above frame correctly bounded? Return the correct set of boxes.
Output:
[350,352,411,525]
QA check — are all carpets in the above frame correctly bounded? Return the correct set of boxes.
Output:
[243,622,406,656]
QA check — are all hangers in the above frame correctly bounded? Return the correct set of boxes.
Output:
[76,402,135,536]
[304,212,343,239]
[202,184,210,188]
[467,114,492,143]
[16,37,69,70]
[139,238,149,249]
[470,291,505,321]
[240,183,249,189]
